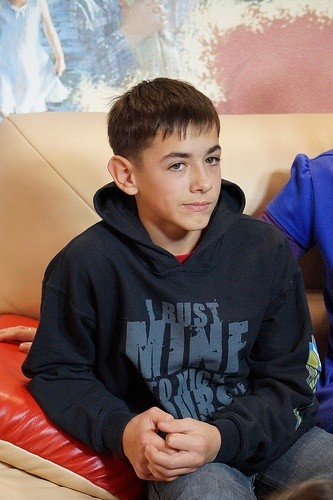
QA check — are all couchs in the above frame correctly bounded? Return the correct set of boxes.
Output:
[0,111,333,500]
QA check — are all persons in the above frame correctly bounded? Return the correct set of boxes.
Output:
[20,76,333,500]
[257,147,333,430]
